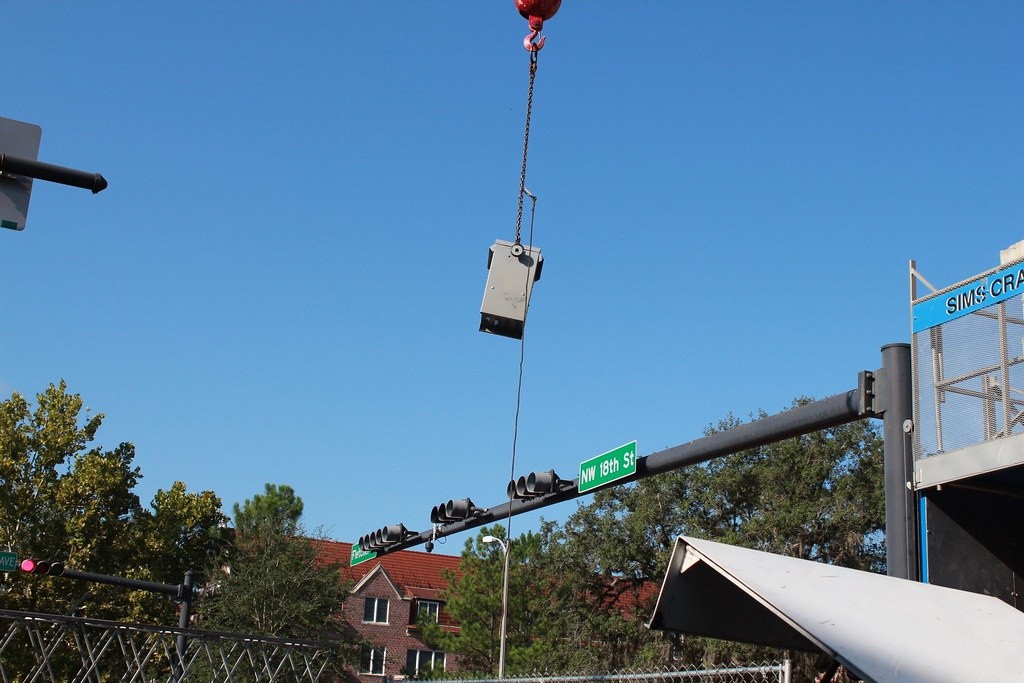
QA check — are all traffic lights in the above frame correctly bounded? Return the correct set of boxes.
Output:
[19,555,65,578]
[507,467,563,499]
[359,523,420,556]
[429,497,488,523]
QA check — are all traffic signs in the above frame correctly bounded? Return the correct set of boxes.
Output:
[350,543,378,567]
[0,551,17,572]
[578,438,638,493]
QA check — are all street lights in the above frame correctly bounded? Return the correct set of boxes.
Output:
[479,534,510,681]
